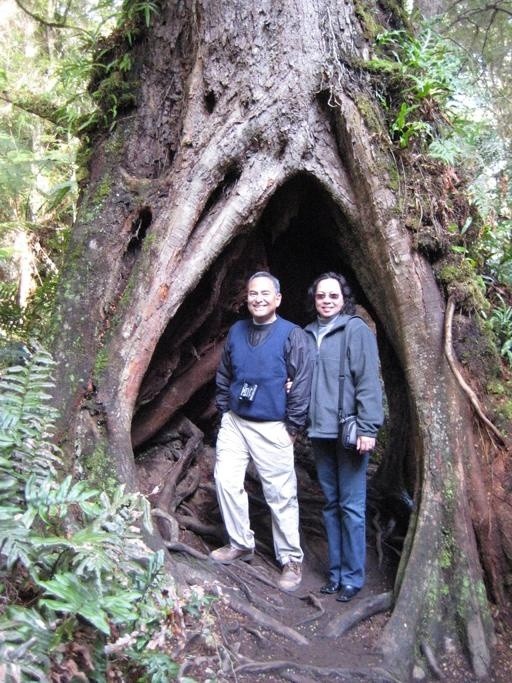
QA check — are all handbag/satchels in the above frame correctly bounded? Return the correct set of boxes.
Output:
[338,415,360,449]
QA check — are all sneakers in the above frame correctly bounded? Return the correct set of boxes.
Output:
[279,559,303,593]
[209,543,255,565]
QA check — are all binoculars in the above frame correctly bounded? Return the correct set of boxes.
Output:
[238,382,258,402]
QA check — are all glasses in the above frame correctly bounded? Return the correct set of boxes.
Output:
[316,291,340,299]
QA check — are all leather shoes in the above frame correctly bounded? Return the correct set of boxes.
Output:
[320,579,341,594]
[336,584,360,602]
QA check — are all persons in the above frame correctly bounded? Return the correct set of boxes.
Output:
[206,270,317,592]
[284,270,384,602]
